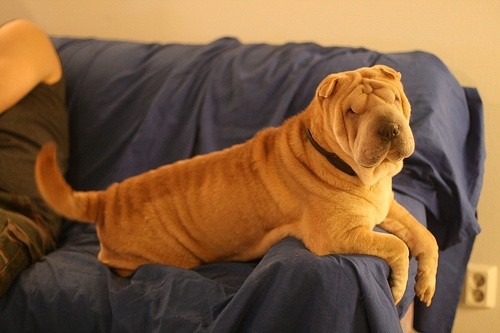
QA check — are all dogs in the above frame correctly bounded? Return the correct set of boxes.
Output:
[32,64,439,308]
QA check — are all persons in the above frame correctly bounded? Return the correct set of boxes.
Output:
[0,11,71,310]
